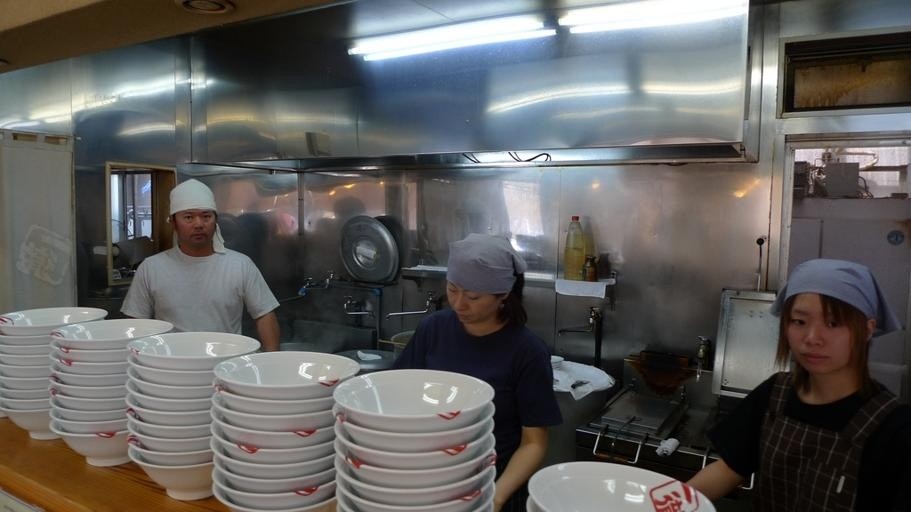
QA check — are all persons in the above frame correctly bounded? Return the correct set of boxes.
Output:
[685,259,911,511]
[119,177,281,356]
[388,231,563,512]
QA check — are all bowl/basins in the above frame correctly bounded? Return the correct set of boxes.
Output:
[210,349,361,511]
[125,331,263,503]
[48,317,175,467]
[0,305,109,443]
[551,355,564,369]
[524,461,717,512]
[333,370,500,511]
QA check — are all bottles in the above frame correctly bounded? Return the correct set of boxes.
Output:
[692,337,709,368]
[565,215,585,282]
[577,270,585,281]
[583,256,598,282]
[597,253,611,279]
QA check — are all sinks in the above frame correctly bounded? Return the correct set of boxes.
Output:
[278,319,378,353]
[657,399,719,452]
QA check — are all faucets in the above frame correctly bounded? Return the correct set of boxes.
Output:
[343,295,375,319]
[297,277,330,296]
[696,336,711,361]
[588,306,599,325]
[384,289,441,319]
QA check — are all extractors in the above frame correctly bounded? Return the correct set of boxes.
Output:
[175,1,762,173]
